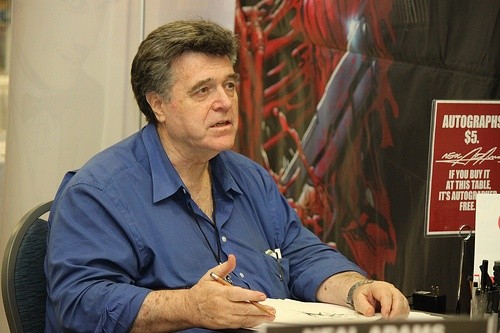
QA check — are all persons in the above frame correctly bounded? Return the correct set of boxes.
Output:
[43,17,410,333]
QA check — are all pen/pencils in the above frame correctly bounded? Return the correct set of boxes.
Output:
[210,272,276,317]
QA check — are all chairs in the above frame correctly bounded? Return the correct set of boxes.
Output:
[1,199,54,333]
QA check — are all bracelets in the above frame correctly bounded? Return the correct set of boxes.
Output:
[346,279,374,312]
